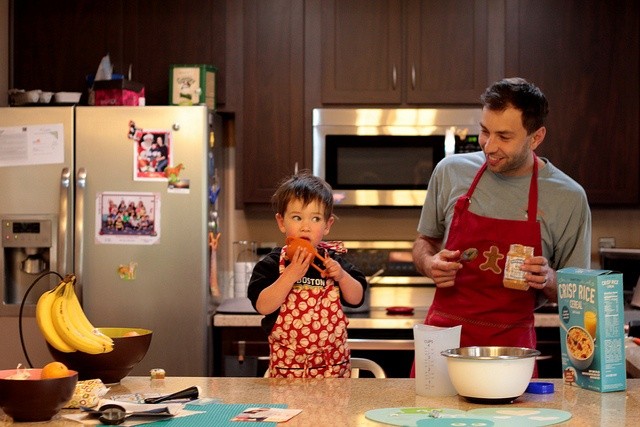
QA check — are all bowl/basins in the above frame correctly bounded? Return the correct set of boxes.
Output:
[440,346,541,404]
[0,369,78,422]
[46,327,153,385]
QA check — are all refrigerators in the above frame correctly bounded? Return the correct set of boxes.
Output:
[0,105,224,377]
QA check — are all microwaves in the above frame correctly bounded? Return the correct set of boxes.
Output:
[313,107,483,207]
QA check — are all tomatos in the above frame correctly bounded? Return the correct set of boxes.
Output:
[287,239,315,264]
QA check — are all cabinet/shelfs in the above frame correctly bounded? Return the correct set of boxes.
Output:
[10,2,227,110]
[306,0,506,106]
[507,1,638,207]
[227,0,305,208]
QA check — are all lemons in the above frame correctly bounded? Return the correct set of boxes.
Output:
[40,361,69,380]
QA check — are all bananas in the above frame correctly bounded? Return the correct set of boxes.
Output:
[38,273,115,356]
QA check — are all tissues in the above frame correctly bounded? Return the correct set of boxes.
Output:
[89,52,146,106]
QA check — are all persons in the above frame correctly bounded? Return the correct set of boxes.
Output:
[105,198,154,234]
[407,76,593,378]
[139,132,169,172]
[246,171,368,380]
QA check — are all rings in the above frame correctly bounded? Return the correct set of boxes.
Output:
[544,276,547,283]
